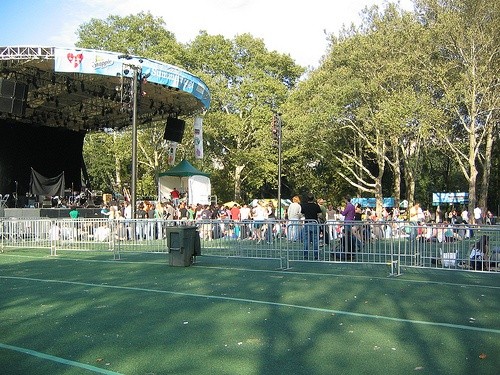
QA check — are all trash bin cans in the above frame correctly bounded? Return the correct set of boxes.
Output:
[167,226,200,268]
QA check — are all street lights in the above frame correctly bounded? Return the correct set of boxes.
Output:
[269,108,282,220]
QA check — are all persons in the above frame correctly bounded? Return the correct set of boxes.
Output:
[337,195,355,261]
[301,194,322,261]
[468,235,500,271]
[49,187,496,244]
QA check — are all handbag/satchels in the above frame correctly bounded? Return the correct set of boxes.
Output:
[470,255,482,270]
[440,248,457,267]
